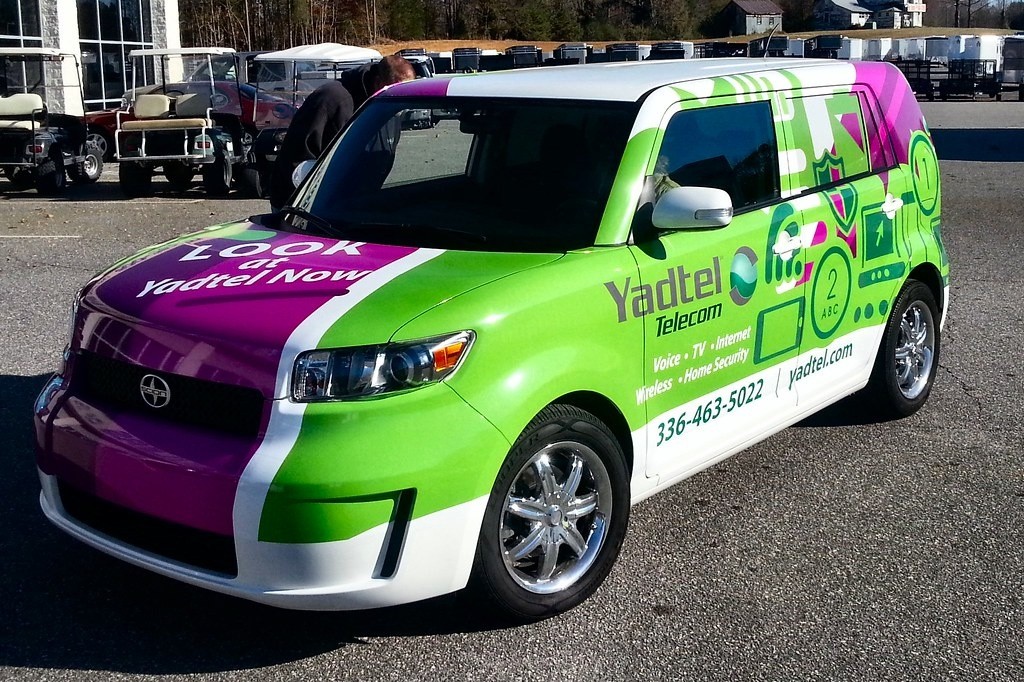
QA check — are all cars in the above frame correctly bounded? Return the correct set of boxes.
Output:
[80,81,304,154]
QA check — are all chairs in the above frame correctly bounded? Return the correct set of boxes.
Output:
[489,124,591,207]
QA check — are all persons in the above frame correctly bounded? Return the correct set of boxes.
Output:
[592,115,682,201]
[269,55,416,213]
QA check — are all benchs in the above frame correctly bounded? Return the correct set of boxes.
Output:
[0,93,43,133]
[121,92,216,131]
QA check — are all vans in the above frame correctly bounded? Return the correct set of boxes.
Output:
[25,56,953,613]
